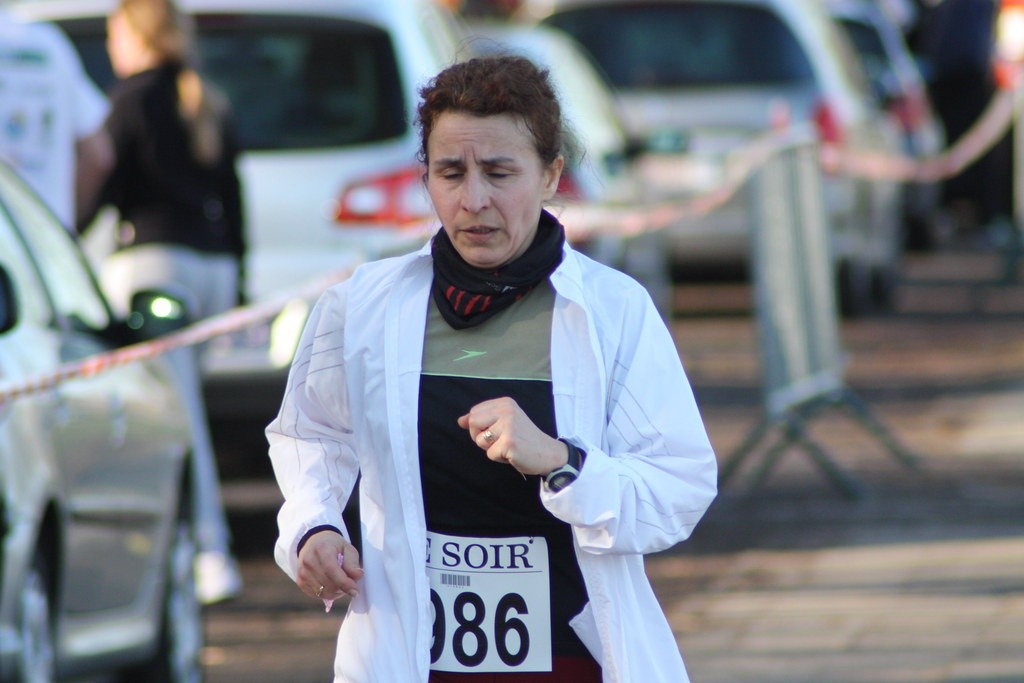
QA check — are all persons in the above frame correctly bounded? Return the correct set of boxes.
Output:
[909,0,1009,223]
[80,0,247,607]
[265,57,717,682]
[0,0,114,242]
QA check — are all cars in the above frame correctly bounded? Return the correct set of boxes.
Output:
[0,0,1024,428]
[1,155,215,681]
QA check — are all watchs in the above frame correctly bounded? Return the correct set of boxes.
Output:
[544,438,580,492]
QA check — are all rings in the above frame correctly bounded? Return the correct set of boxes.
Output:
[483,430,493,444]
[316,586,323,597]
